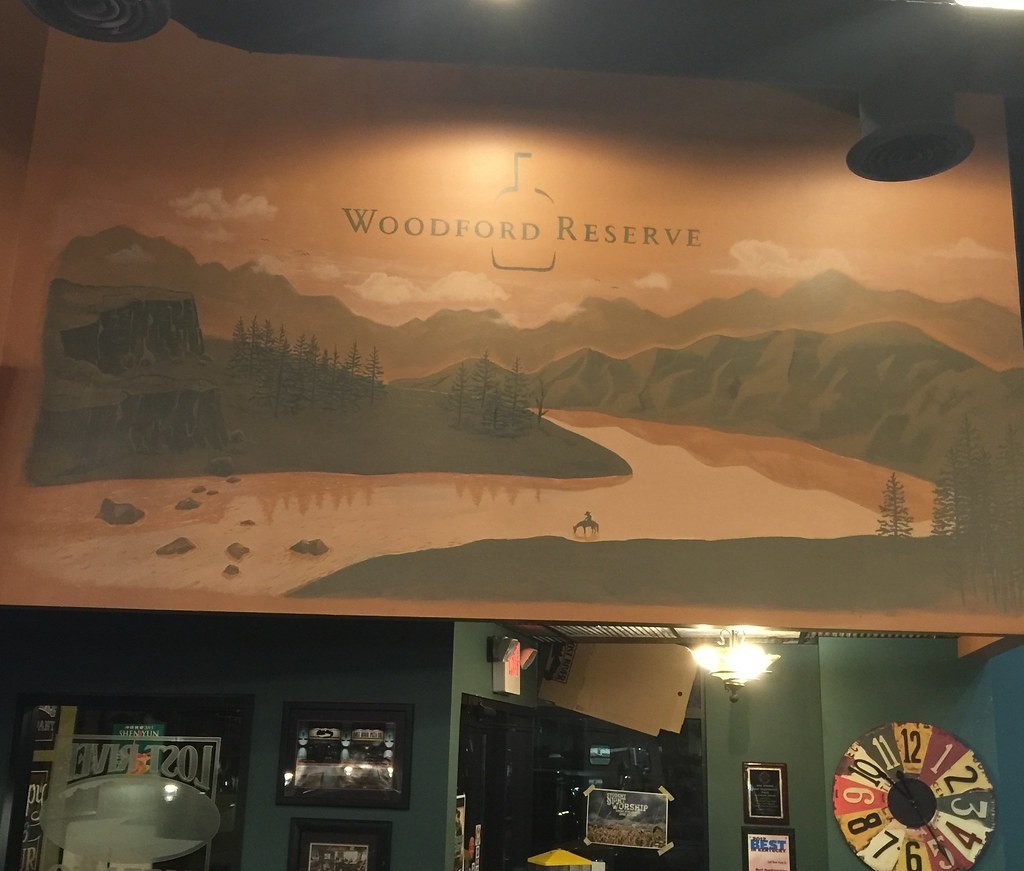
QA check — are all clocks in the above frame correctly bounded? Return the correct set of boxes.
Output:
[830,721,996,871]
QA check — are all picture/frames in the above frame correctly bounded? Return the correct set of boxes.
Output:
[742,824,797,871]
[275,700,414,809]
[287,816,393,871]
[742,761,790,825]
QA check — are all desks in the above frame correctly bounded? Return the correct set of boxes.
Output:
[37,773,221,871]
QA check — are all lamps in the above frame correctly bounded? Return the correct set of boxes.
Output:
[684,628,783,704]
